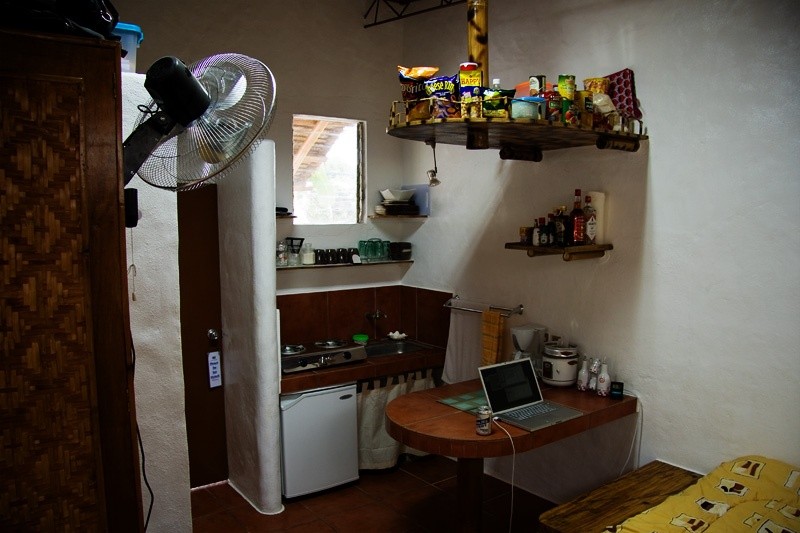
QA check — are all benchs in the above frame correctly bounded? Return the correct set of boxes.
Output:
[537,461,709,532]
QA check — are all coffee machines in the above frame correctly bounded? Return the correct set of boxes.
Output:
[510,323,549,383]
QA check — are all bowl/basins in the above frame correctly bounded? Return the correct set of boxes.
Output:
[512,98,539,119]
[379,188,416,201]
[391,242,412,260]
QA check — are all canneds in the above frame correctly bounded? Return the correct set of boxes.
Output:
[475,406,491,435]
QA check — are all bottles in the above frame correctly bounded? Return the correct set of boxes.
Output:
[482,78,506,117]
[276,242,315,267]
[576,358,611,396]
[521,189,596,247]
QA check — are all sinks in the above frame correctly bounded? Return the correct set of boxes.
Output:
[364,337,434,357]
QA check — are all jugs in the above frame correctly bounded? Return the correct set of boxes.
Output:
[514,351,538,376]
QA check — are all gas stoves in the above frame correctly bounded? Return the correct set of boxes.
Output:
[281,338,369,374]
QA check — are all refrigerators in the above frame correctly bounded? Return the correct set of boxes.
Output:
[281,381,361,498]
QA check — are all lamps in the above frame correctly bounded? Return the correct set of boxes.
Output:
[425,137,441,188]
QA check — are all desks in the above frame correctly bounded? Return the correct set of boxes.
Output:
[385,379,638,533]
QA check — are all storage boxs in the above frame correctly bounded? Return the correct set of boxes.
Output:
[112,23,144,75]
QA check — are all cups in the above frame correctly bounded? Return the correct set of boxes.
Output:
[314,248,359,263]
[529,74,609,130]
[359,239,391,262]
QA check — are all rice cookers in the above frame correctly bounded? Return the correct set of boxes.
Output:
[541,343,582,387]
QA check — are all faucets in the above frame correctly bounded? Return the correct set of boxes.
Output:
[368,308,388,320]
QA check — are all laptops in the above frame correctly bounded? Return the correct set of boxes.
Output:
[477,356,585,433]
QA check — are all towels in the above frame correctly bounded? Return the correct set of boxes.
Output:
[481,311,504,367]
[442,299,494,384]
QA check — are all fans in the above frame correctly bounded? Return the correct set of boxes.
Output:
[122,52,276,229]
